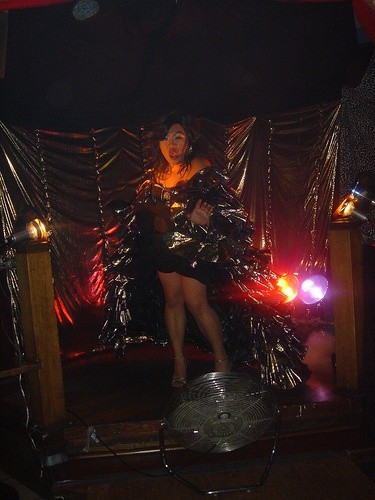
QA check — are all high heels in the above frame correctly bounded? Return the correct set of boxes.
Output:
[213,353,233,373]
[171,355,187,391]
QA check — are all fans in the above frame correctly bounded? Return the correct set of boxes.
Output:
[157,369,283,496]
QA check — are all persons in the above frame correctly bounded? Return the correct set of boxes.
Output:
[135,115,257,389]
